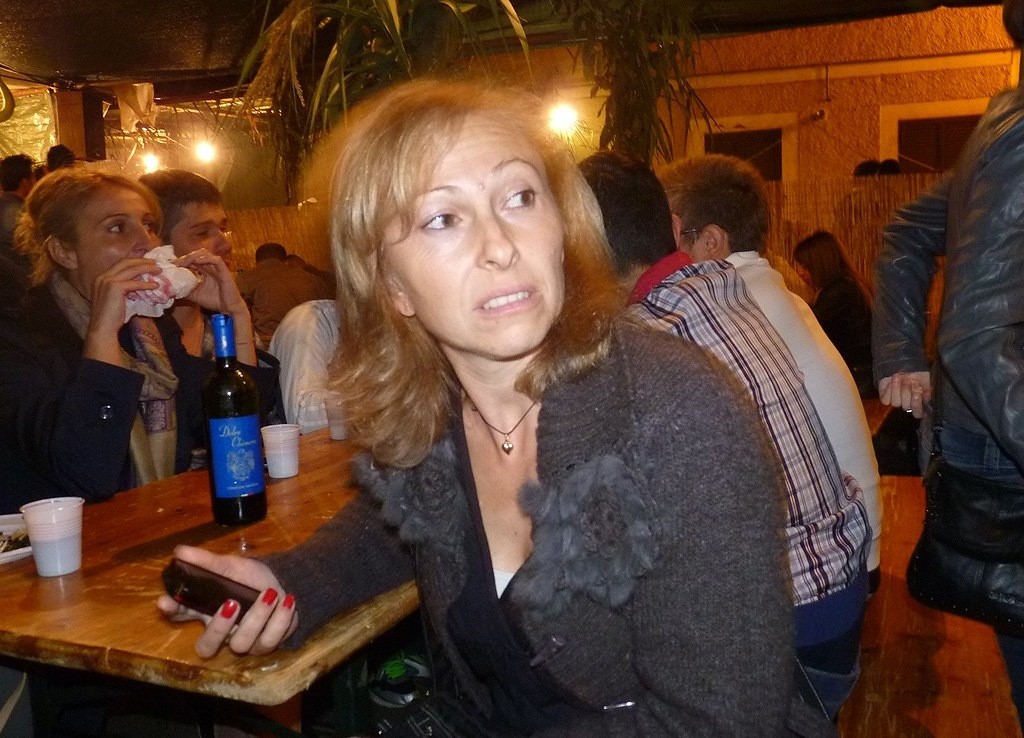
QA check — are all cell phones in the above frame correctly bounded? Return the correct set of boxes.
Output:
[162,558,260,624]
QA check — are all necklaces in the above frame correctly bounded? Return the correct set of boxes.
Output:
[471,400,537,455]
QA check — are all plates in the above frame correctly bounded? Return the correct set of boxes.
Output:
[0,514,31,565]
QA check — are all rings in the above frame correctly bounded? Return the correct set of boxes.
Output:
[907,410,912,412]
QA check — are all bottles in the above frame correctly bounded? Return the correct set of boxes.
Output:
[205,314,267,526]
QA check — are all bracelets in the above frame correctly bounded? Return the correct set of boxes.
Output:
[236,338,256,345]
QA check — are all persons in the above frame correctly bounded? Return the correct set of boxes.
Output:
[147,168,238,474]
[576,149,873,649]
[793,231,876,399]
[576,126,677,274]
[233,242,331,350]
[267,299,339,435]
[871,0,1024,717]
[35,144,75,182]
[657,153,884,592]
[0,166,286,516]
[0,154,36,239]
[155,81,833,738]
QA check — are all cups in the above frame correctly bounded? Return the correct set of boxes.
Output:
[20,497,85,577]
[324,397,348,440]
[261,424,301,478]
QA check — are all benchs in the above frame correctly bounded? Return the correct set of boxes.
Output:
[836,477,1024,738]
[860,396,900,436]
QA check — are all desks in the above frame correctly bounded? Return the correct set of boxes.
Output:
[0,429,422,738]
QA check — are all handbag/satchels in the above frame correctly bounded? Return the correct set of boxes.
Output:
[908,458,1024,638]
